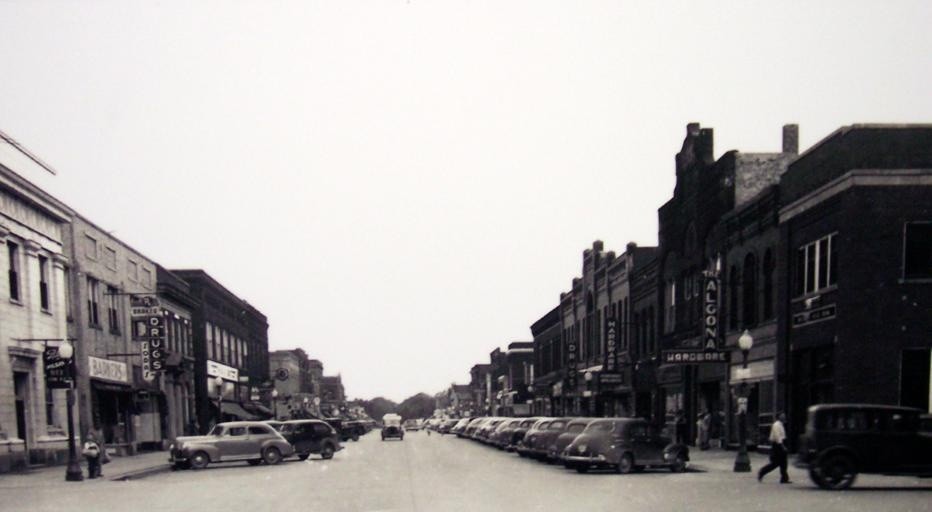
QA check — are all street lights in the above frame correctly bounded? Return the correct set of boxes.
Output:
[451,384,533,418]
[304,396,364,419]
[213,375,223,435]
[730,328,752,473]
[584,370,592,415]
[58,338,83,482]
[271,388,279,420]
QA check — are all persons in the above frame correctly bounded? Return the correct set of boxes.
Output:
[695,408,711,450]
[81,421,105,479]
[426,420,431,435]
[439,419,445,436]
[757,412,793,483]
[675,410,687,444]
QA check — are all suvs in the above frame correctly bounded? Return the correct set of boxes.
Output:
[380,414,405,441]
[793,404,932,490]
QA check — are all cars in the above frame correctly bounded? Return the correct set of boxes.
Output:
[253,415,378,461]
[404,419,420,431]
[168,421,294,469]
[421,416,692,476]
